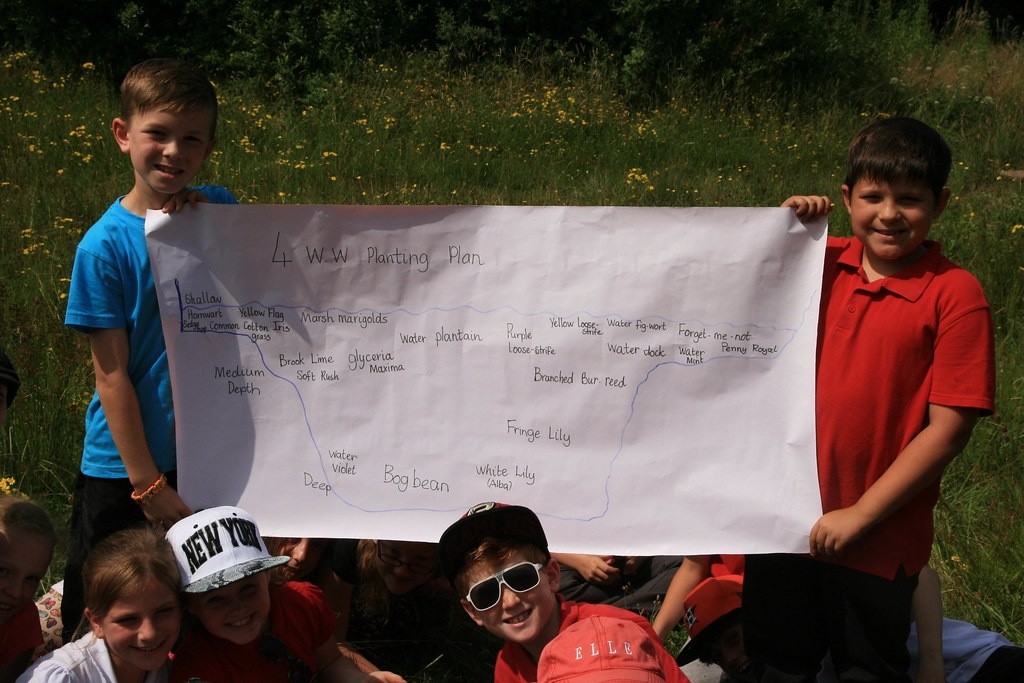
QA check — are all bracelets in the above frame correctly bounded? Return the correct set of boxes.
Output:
[130,474,167,504]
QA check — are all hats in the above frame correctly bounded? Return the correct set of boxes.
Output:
[439,502,548,579]
[165,506,290,592]
[676,575,744,666]
[537,614,666,683]
[0,348,21,410]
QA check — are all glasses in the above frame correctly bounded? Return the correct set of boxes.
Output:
[464,561,547,611]
[377,540,435,575]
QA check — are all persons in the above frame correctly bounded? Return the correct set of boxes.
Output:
[59,55,244,641]
[740,114,996,683]
[0,489,1024,683]
